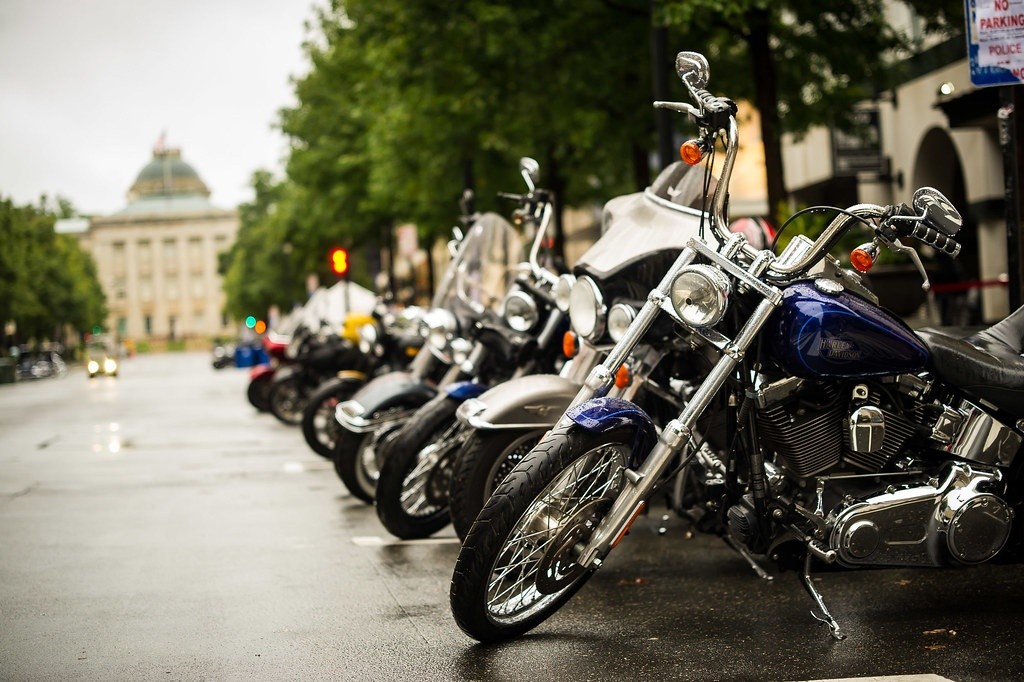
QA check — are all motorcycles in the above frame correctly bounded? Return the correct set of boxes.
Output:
[245,49,1024,651]
